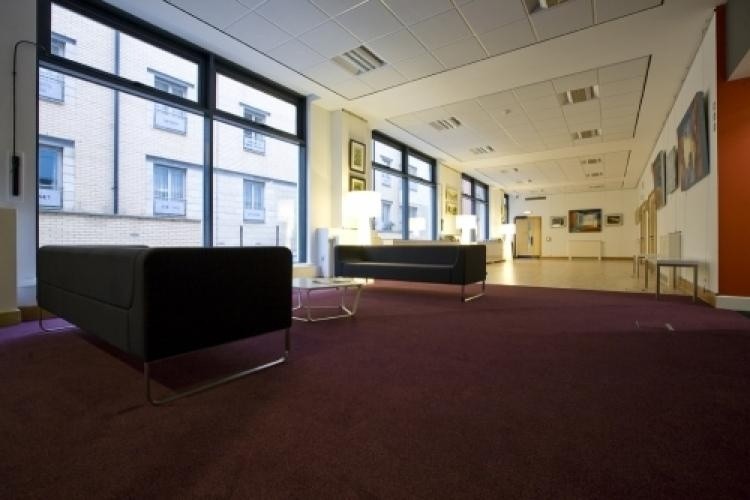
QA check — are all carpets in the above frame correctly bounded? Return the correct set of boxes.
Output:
[0,281,750,499]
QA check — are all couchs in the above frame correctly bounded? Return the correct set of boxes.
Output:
[334,242,487,304]
[33,242,294,408]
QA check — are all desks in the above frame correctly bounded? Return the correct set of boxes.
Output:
[566,238,604,262]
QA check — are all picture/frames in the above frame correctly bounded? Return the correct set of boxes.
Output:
[348,138,367,191]
